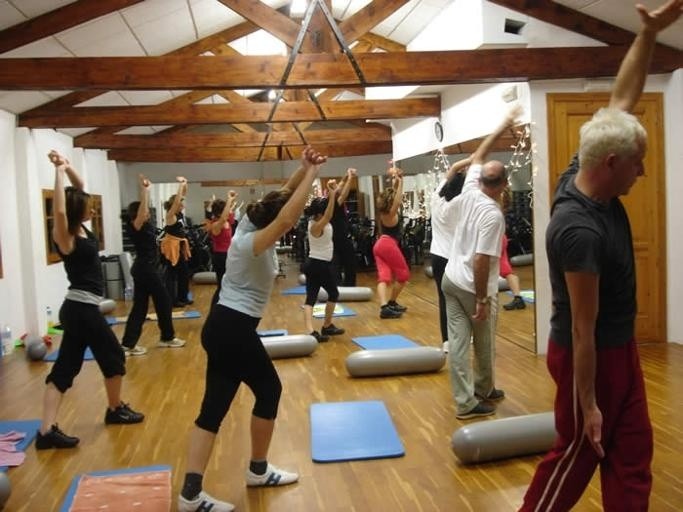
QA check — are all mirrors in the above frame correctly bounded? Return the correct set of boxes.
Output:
[393,122,539,356]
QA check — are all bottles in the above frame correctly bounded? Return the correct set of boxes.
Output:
[124,283,133,304]
[47,306,54,328]
[0,325,11,357]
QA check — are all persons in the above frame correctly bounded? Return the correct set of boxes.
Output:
[35,146,143,450]
[429,153,472,355]
[440,104,527,420]
[516,0,682,511]
[176,141,329,511]
[500,235,524,312]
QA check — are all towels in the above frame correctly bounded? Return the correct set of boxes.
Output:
[115,309,184,323]
[68,468,172,511]
[0,427,29,466]
[309,303,344,314]
[514,288,535,301]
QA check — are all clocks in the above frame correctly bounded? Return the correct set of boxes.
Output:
[433,120,444,142]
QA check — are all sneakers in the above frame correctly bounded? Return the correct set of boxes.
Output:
[244,461,300,487]
[310,330,330,342]
[442,340,449,354]
[120,343,147,356]
[35,422,80,449]
[502,298,526,311]
[388,302,408,312]
[156,336,186,348]
[474,387,505,403]
[320,322,346,335]
[454,403,498,420]
[379,305,403,318]
[177,490,237,512]
[174,298,193,308]
[104,400,145,424]
